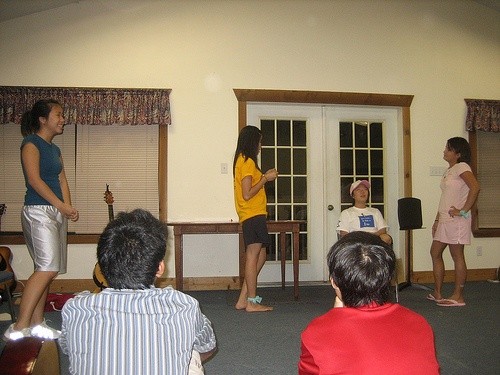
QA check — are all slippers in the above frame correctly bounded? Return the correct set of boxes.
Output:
[436,297,468,308]
[426,292,442,302]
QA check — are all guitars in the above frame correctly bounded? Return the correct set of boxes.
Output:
[93,184,115,288]
[0,204,17,290]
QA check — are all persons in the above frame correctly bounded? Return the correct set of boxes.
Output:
[425,137,481,307]
[233,126,278,312]
[57,209,218,375]
[2,99,79,340]
[298,231,441,375]
[336,180,393,250]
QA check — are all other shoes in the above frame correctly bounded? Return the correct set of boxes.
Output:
[2,317,63,346]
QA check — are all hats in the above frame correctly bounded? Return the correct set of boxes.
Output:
[349,179,371,196]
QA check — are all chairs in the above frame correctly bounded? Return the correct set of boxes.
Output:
[0,271,16,325]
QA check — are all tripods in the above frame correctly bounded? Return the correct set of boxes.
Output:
[391,197,433,294]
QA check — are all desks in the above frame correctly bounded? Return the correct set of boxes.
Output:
[167,219,309,301]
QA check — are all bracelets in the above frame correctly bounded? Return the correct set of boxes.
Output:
[459,208,469,219]
[435,220,439,221]
[263,175,268,183]
